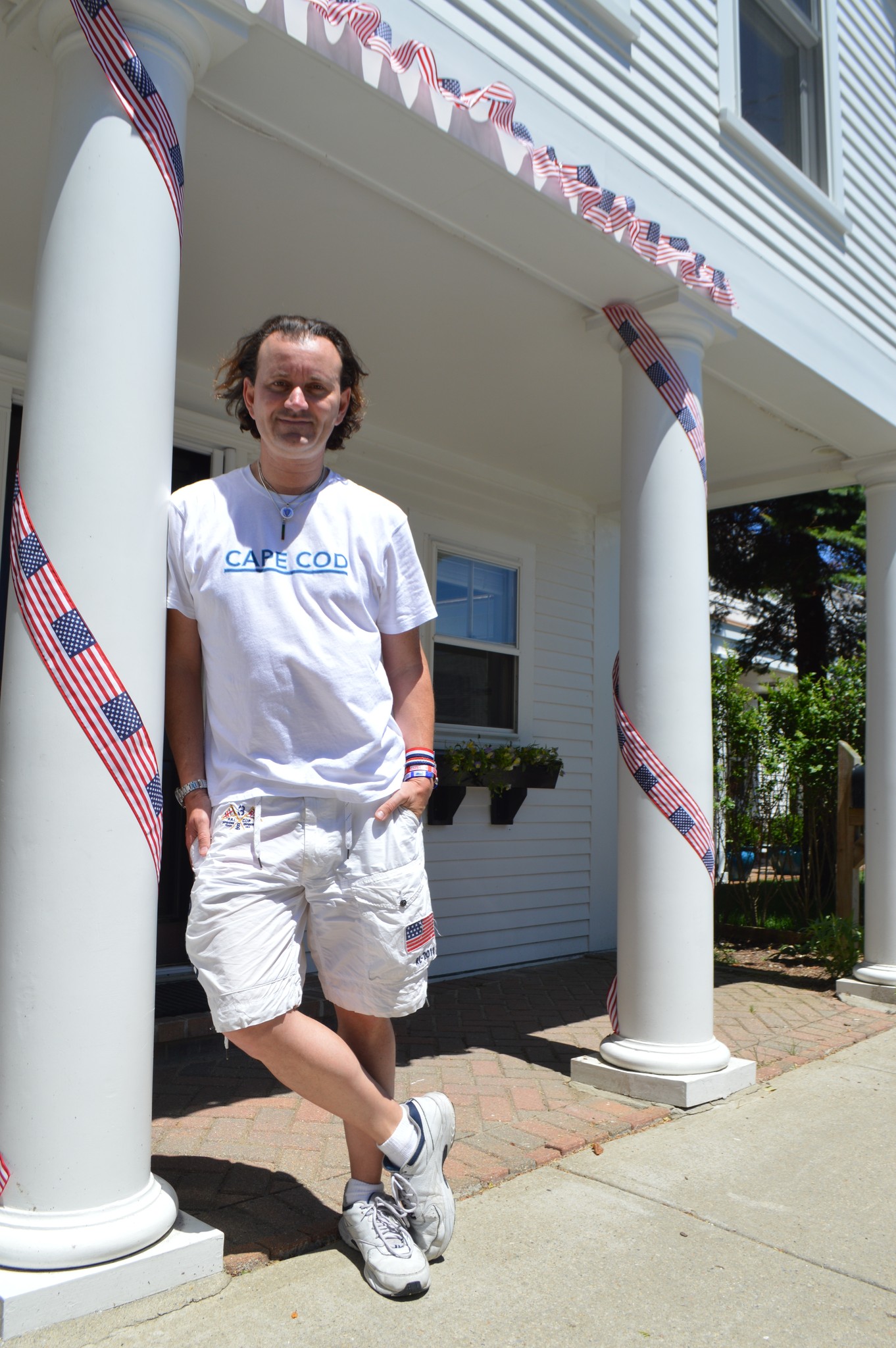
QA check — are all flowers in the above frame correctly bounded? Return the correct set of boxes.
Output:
[429,739,559,774]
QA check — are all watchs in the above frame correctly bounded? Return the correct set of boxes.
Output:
[175,780,207,810]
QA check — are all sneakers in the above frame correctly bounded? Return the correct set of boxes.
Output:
[337,1192,431,1299]
[383,1091,455,1259]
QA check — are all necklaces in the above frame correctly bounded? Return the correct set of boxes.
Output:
[258,460,323,520]
[257,462,325,539]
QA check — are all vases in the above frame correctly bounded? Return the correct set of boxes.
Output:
[412,770,560,828]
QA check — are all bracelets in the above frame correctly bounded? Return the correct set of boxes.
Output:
[404,747,438,789]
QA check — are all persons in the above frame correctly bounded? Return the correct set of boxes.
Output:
[168,317,462,1302]
